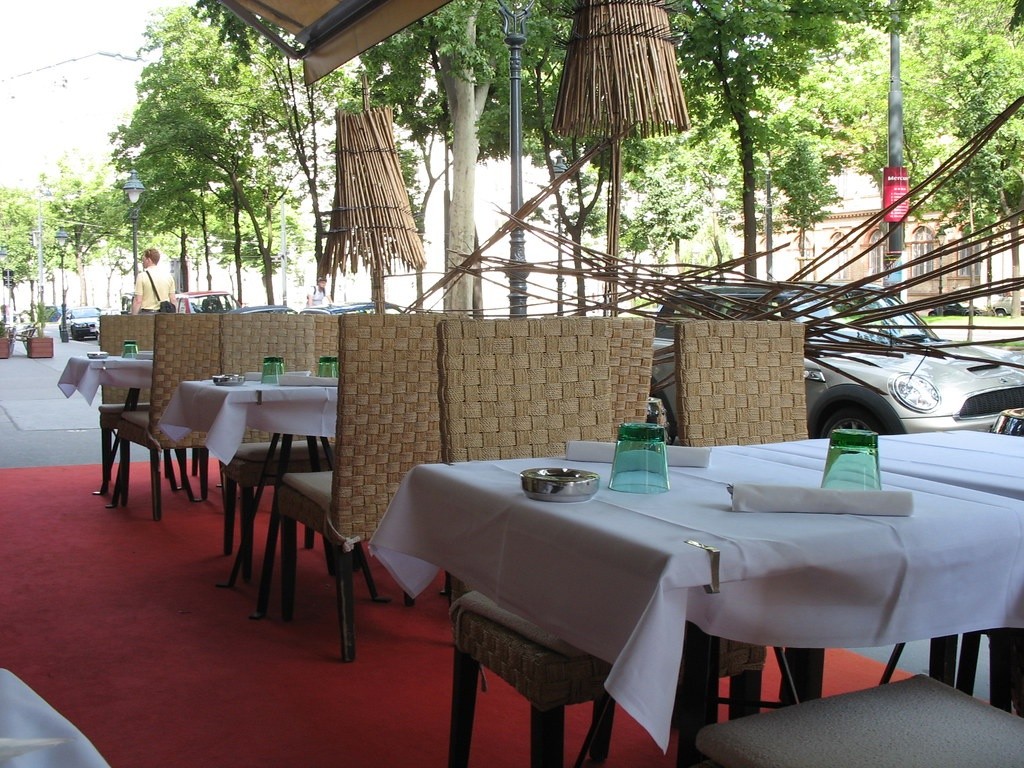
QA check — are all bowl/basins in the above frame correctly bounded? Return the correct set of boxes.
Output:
[244,372,261,380]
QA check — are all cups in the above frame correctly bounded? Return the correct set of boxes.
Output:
[821,429,882,492]
[123,344,138,357]
[319,356,339,379]
[121,340,136,356]
[261,356,285,384]
[608,422,670,494]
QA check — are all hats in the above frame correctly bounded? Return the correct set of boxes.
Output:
[318,276,327,282]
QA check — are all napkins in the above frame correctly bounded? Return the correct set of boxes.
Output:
[138,350,153,353]
[280,374,337,386]
[245,371,311,381]
[565,440,712,467]
[726,482,914,517]
[136,353,154,360]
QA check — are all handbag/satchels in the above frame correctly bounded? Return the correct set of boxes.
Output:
[160,301,176,313]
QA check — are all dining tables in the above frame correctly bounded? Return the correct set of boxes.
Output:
[368,428,1024,768]
[158,371,338,619]
[57,351,153,510]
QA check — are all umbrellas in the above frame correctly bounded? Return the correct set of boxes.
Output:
[316,74,427,312]
[550,0,691,316]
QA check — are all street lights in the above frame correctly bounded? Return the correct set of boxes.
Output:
[123,169,145,286]
[36,186,52,304]
[553,156,565,317]
[0,245,11,324]
[55,227,69,342]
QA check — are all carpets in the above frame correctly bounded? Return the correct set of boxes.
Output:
[0,457,916,768]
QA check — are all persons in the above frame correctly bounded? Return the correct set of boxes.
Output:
[303,277,333,310]
[131,249,178,315]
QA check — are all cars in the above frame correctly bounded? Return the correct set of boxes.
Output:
[299,301,404,316]
[645,282,1024,439]
[229,305,297,316]
[174,290,240,315]
[961,293,1024,316]
[928,302,983,316]
[58,307,102,341]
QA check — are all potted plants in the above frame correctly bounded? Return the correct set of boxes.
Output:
[27,299,57,358]
[0,304,12,359]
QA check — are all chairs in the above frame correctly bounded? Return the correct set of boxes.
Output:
[202,298,223,313]
[312,315,342,374]
[674,319,958,714]
[118,313,226,523]
[433,317,702,768]
[698,674,1024,768]
[279,312,469,663]
[98,315,177,495]
[217,314,334,557]
[541,317,655,442]
